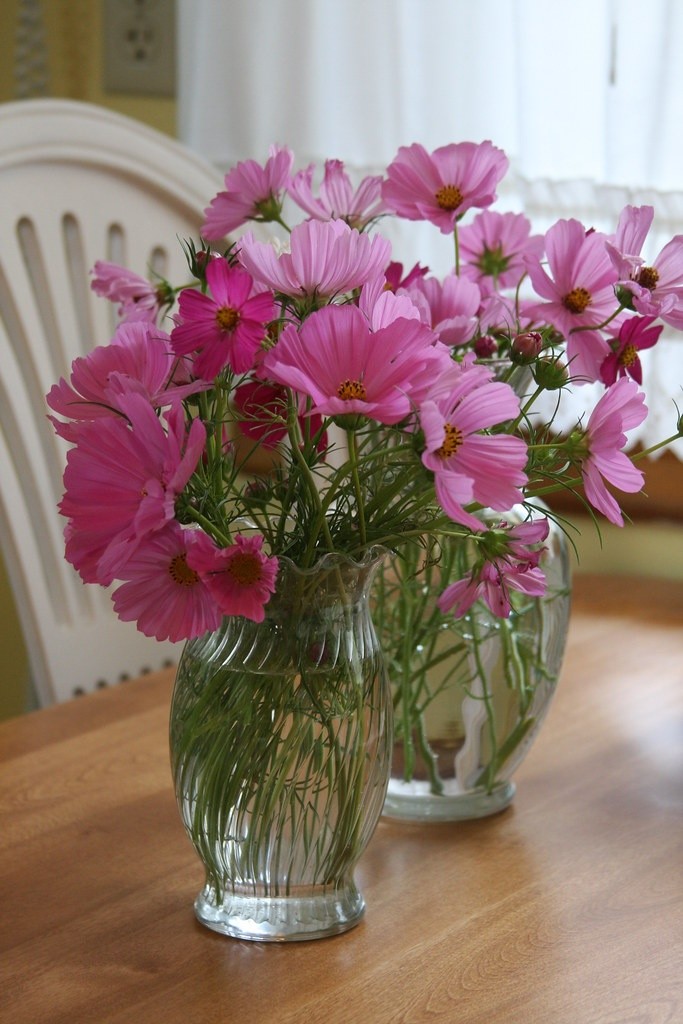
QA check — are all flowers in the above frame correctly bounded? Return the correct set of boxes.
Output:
[42,140,683,909]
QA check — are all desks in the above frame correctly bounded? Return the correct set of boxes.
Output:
[0,566,683,1024]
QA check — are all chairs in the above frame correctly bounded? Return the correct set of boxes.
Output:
[0,98,253,708]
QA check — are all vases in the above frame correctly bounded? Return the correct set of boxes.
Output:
[368,487,569,825]
[170,512,393,944]
